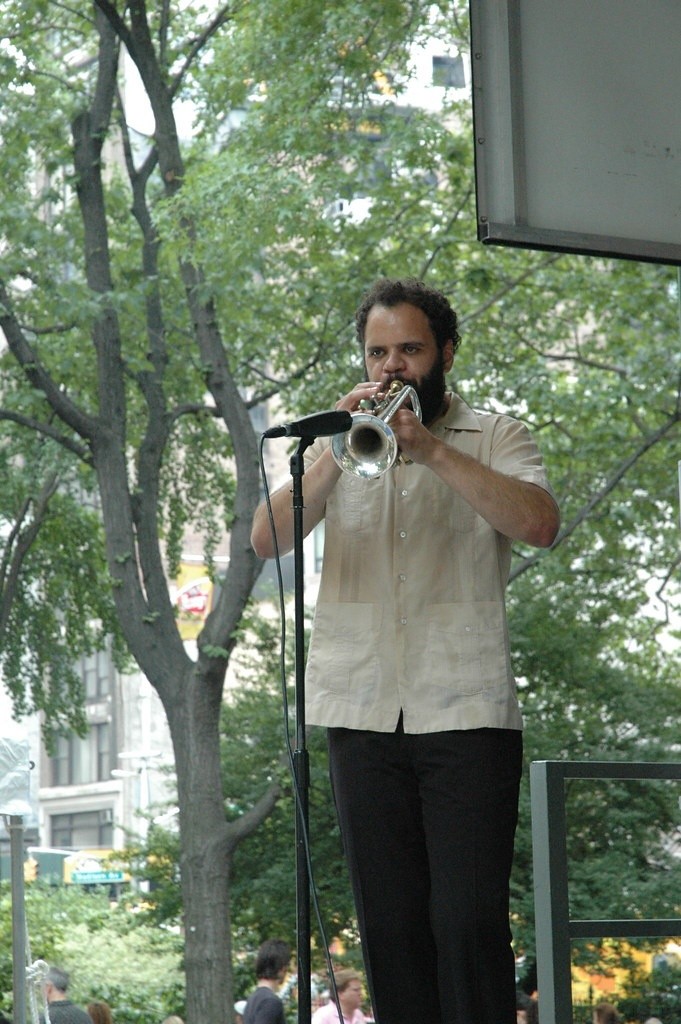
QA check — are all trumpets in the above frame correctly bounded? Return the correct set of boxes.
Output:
[329,378,424,482]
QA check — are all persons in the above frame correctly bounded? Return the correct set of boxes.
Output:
[32,968,93,1024]
[311,967,367,1024]
[250,280,560,1024]
[161,1015,184,1024]
[88,1002,112,1024]
[592,1003,618,1024]
[233,1000,247,1024]
[644,1018,663,1024]
[243,941,289,1024]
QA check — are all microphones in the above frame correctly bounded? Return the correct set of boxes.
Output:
[263,410,353,438]
